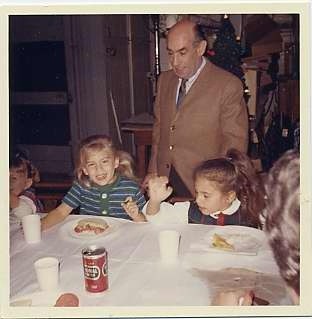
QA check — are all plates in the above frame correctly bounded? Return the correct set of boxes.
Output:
[204,224,269,253]
[60,218,119,240]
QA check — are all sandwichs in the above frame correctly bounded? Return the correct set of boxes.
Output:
[213,233,232,251]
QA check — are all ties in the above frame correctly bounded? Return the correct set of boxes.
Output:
[178,79,187,103]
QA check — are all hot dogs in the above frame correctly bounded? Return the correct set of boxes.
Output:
[74,217,108,235]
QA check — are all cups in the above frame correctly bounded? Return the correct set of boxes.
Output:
[34,257,60,297]
[22,215,41,247]
[159,231,179,262]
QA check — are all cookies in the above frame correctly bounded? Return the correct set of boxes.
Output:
[55,293,79,308]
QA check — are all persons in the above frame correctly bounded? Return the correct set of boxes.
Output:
[142,17,249,204]
[20,136,146,235]
[264,149,301,304]
[9,152,41,232]
[142,148,266,230]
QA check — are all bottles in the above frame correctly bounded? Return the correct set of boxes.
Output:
[81,245,110,293]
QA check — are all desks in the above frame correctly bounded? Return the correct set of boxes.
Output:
[122,123,153,179]
